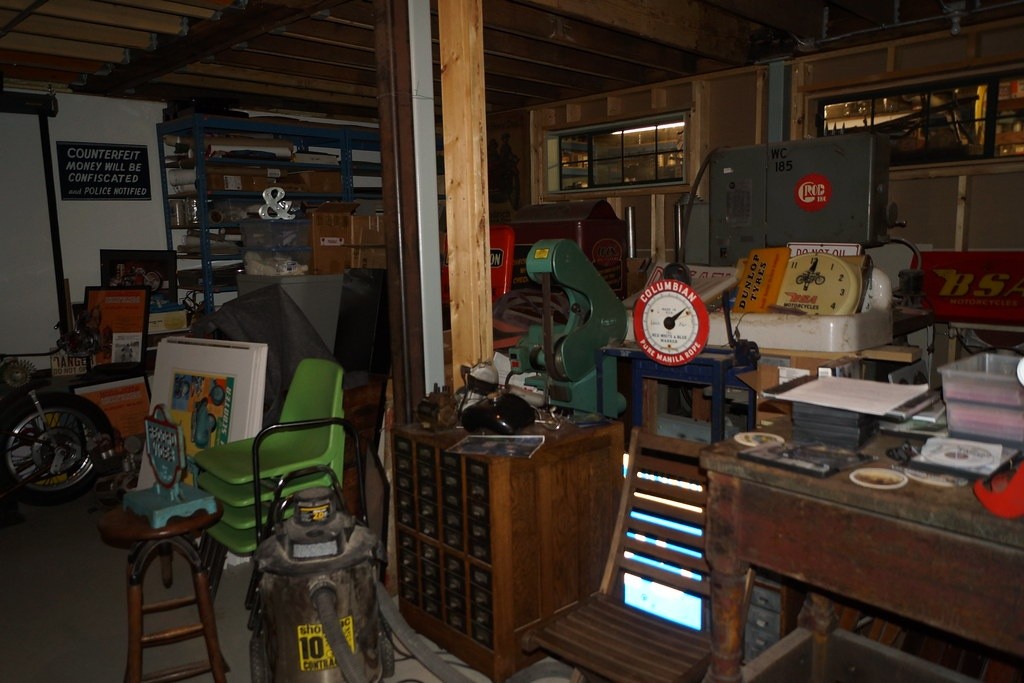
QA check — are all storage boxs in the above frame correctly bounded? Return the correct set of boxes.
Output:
[240,220,309,247]
[351,214,386,246]
[308,200,360,245]
[149,312,187,333]
[938,350,1024,451]
[286,172,341,193]
[311,247,346,275]
[207,175,268,192]
[240,248,311,275]
[624,308,894,354]
[351,247,385,268]
[212,197,265,223]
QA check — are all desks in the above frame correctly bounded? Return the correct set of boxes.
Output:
[697,428,1024,683]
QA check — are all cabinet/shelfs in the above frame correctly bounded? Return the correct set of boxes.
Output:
[392,417,624,683]
[152,118,447,324]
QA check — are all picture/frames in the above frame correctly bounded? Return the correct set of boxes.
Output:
[82,285,150,367]
[67,373,151,486]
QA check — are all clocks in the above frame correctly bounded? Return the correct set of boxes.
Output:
[775,251,858,314]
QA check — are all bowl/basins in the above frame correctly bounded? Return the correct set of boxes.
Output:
[238,219,312,276]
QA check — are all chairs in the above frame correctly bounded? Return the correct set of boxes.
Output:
[196,358,347,631]
[518,427,751,683]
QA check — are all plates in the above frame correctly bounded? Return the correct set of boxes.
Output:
[903,466,969,488]
[848,467,908,490]
[734,430,785,448]
[921,443,994,468]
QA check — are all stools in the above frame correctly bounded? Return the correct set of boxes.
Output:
[99,501,231,683]
